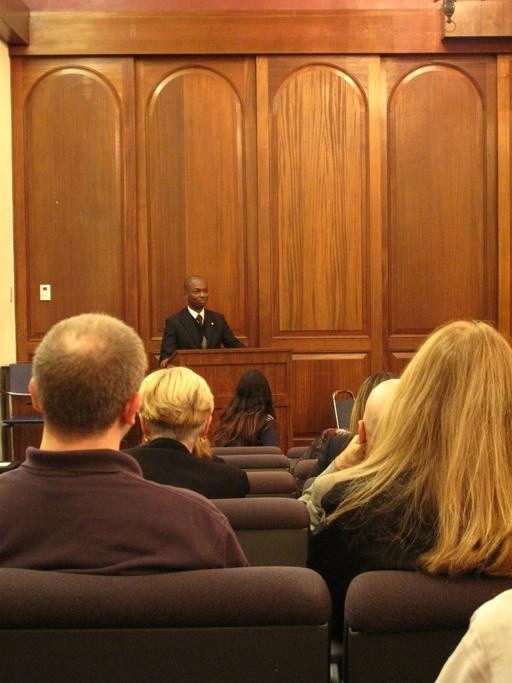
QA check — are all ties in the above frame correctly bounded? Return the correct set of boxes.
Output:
[196,314,204,344]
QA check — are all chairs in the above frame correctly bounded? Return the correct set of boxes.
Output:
[0,363,47,461]
[332,390,356,430]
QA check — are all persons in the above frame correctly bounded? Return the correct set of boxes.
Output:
[124,365,251,496]
[209,368,280,446]
[301,315,512,683]
[1,310,248,571]
[160,272,249,363]
